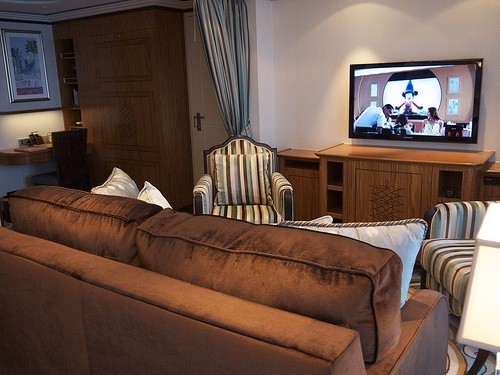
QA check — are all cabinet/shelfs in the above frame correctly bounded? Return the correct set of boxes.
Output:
[53,9,193,211]
[279,143,500,223]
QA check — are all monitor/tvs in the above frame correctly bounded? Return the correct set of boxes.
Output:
[347,57,483,152]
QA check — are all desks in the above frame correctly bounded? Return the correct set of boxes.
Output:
[0,142,92,166]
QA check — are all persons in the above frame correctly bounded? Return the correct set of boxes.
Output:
[393,114,413,134]
[421,107,443,133]
[355,104,394,130]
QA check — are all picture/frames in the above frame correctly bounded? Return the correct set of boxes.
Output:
[0,29,51,104]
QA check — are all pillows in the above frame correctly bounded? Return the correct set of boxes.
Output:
[277,217,427,308]
[137,181,173,210]
[212,153,273,206]
[90,167,139,198]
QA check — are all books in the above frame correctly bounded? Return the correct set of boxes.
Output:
[14,146,48,152]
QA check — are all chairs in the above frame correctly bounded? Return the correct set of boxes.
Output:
[193,135,294,225]
[33,128,87,190]
[418,200,500,317]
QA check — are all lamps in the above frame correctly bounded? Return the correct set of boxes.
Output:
[456,202,500,353]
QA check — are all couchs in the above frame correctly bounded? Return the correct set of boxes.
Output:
[1,187,450,375]
